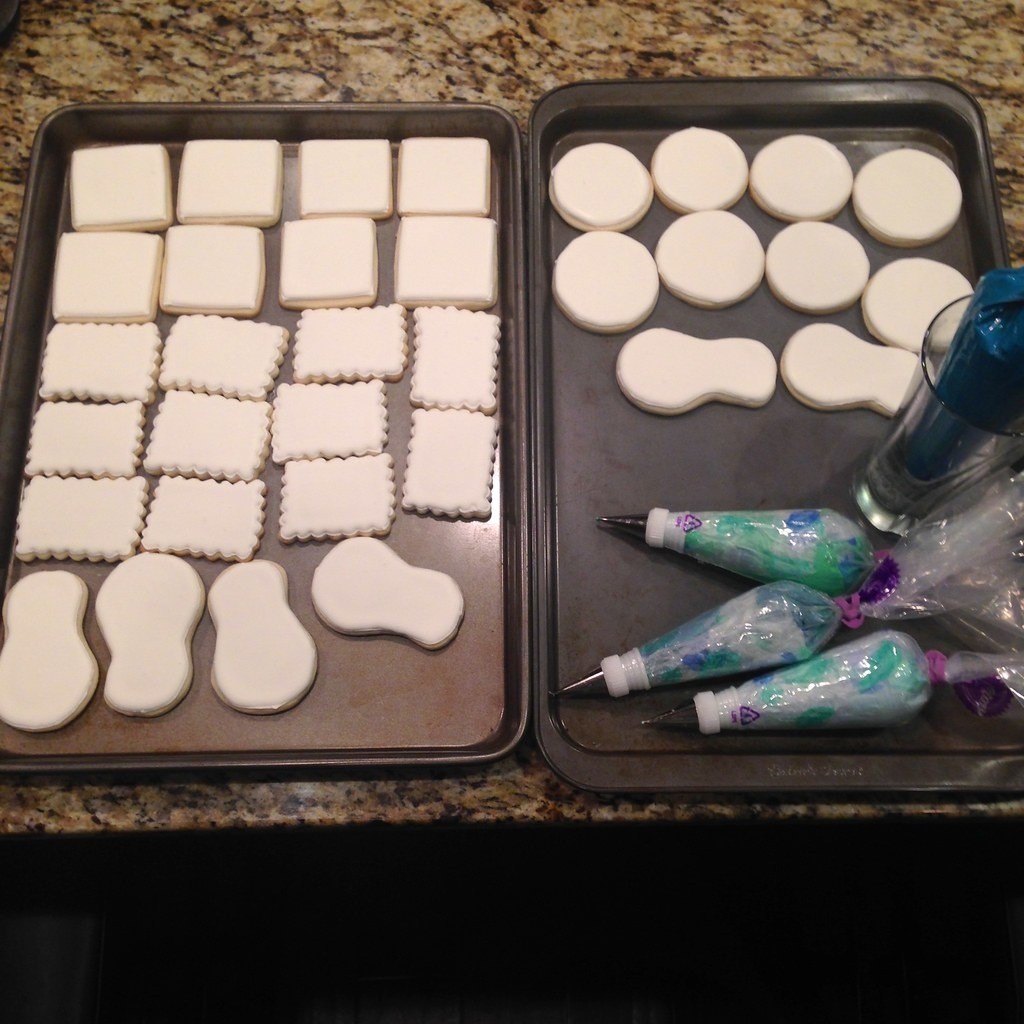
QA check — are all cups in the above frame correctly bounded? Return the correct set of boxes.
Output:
[848,291,1024,536]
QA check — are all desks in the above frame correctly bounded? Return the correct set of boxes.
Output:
[0,0,1024,845]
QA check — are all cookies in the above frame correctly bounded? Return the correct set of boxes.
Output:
[548,126,976,417]
[0,135,501,732]
